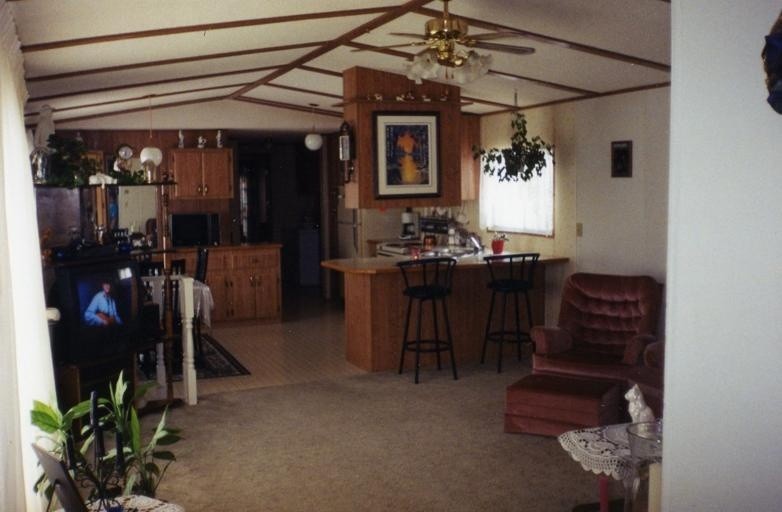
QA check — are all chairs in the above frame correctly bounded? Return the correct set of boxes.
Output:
[195,242,210,285]
[525,268,663,398]
[478,248,543,376]
[395,254,462,387]
[139,272,187,383]
[136,258,187,278]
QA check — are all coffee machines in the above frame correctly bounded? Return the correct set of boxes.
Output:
[398,212,421,240]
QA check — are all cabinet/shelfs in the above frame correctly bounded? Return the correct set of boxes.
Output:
[169,244,230,325]
[50,343,184,444]
[167,145,238,204]
[229,240,283,329]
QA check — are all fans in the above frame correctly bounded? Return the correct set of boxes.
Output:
[346,0,539,60]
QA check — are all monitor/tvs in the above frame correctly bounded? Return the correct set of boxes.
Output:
[56,260,144,359]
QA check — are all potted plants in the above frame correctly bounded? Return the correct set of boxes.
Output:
[491,230,511,254]
[468,114,558,182]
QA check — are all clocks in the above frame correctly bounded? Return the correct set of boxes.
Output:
[115,143,134,160]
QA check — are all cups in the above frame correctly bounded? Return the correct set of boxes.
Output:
[491,239,504,255]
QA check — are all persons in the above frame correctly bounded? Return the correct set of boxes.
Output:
[83,268,123,327]
[108,190,119,231]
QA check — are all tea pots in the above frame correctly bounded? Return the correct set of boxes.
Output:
[197,136,207,148]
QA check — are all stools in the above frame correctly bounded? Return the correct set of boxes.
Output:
[503,369,627,442]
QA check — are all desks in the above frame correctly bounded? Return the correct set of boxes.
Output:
[555,420,663,511]
[136,273,218,380]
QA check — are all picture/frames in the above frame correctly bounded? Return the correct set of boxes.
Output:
[609,138,634,179]
[369,107,442,202]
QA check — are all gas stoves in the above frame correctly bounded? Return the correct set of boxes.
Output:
[376,241,426,254]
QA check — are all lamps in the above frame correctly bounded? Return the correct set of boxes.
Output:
[304,102,325,153]
[403,40,494,90]
[139,92,164,168]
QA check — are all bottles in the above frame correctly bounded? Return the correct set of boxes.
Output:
[217,130,224,148]
[177,128,185,149]
[624,420,662,512]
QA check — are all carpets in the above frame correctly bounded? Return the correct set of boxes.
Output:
[139,330,252,383]
[124,310,373,403]
